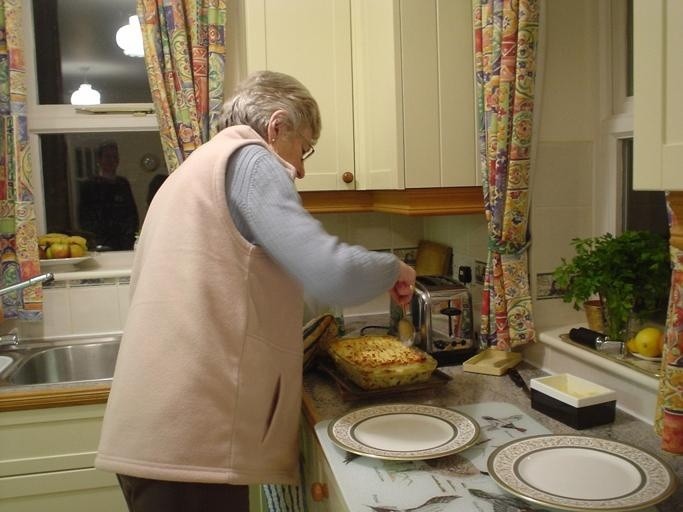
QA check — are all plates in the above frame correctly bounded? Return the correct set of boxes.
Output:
[486,432,677,512]
[39,257,92,265]
[325,401,482,463]
[630,351,663,362]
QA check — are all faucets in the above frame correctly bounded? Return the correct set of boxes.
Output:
[0,335,18,345]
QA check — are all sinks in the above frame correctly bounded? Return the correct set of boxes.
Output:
[9,342,121,386]
[0,350,24,380]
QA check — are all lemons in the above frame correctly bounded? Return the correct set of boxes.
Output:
[633,328,663,356]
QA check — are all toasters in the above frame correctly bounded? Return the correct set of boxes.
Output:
[387,272,476,365]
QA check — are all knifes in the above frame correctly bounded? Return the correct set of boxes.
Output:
[508,367,533,401]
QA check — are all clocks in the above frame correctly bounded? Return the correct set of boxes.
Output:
[138,153,159,173]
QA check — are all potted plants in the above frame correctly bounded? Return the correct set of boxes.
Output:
[551,227,671,342]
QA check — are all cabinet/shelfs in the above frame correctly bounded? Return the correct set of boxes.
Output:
[626,1,683,195]
[351,1,483,192]
[1,402,126,511]
[225,2,356,195]
[297,410,346,511]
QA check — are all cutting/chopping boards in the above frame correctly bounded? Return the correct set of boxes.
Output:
[331,378,434,403]
[414,238,454,278]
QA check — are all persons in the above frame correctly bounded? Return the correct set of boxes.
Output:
[92,69,418,511]
[76,140,140,251]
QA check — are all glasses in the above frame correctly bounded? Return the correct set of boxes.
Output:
[275,119,317,163]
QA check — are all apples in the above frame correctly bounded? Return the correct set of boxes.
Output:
[39,233,87,259]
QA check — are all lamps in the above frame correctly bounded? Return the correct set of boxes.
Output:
[70,63,102,108]
